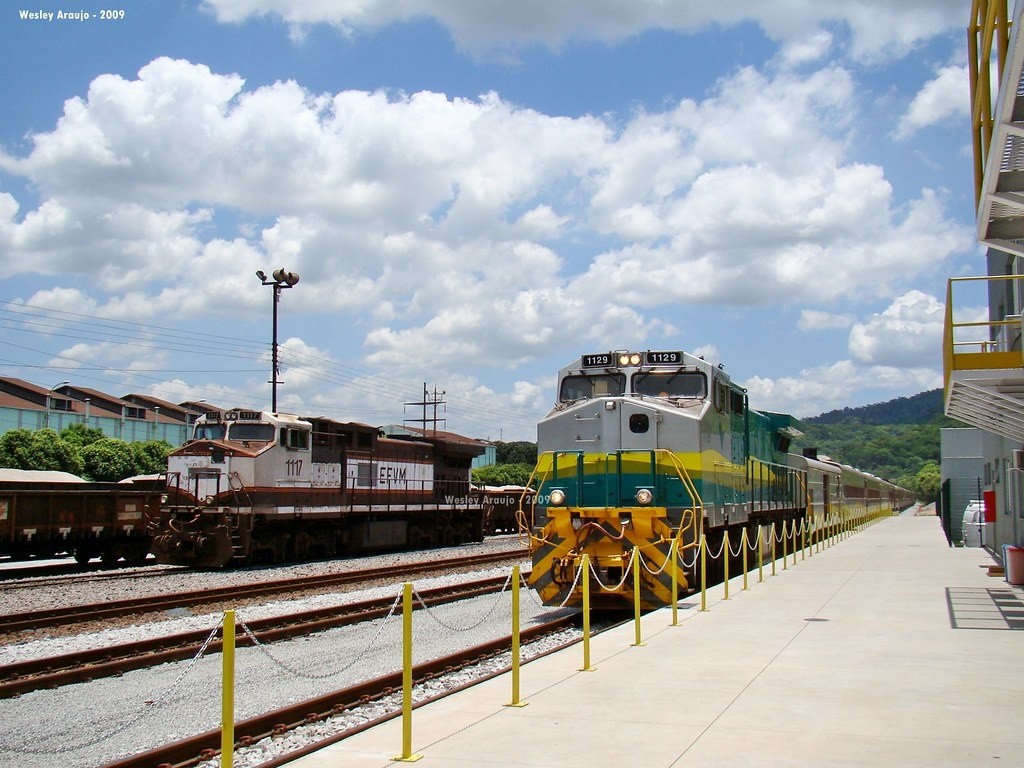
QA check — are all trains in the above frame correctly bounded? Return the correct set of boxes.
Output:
[0,468,538,567]
[135,407,488,571]
[515,347,915,611]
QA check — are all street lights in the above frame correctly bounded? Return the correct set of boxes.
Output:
[255,268,300,412]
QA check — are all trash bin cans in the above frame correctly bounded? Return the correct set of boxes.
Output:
[1002,544,1024,585]
